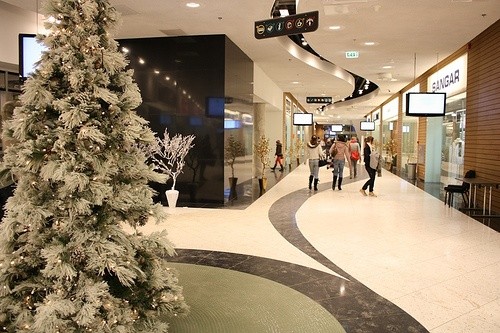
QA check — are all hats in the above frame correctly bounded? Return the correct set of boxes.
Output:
[350,137,357,142]
[276,140,280,144]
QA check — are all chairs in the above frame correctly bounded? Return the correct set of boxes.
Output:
[444,170,476,208]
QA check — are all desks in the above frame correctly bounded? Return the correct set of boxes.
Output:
[456,178,500,217]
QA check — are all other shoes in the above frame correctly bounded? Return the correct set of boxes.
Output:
[369,193,377,197]
[360,189,367,195]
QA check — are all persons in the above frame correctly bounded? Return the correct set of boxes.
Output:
[360,136,382,197]
[303,135,325,191]
[348,137,362,179]
[318,137,335,160]
[329,135,352,190]
[270,140,284,171]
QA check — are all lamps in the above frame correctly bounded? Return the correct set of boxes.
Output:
[365,86,368,89]
[358,91,363,94]
[366,80,370,84]
[302,40,307,45]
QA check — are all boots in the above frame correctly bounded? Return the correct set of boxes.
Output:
[338,177,342,190]
[271,164,276,170]
[309,175,313,189]
[314,178,318,190]
[332,176,337,190]
[279,164,283,171]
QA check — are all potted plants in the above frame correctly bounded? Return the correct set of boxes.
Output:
[226,136,243,201]
[163,131,196,207]
[254,135,271,190]
[187,152,205,193]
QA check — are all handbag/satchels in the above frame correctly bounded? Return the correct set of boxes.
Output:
[331,143,338,157]
[351,151,360,161]
[319,157,327,167]
